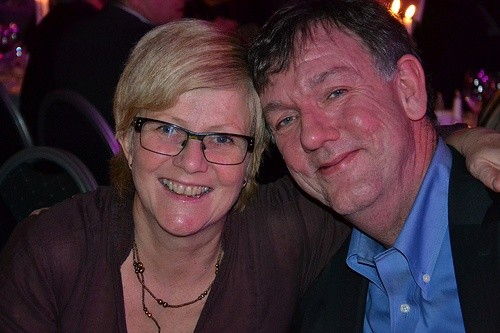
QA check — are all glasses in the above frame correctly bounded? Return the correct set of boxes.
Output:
[131,117,255,165]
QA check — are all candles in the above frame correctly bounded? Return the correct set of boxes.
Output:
[402,5,416,38]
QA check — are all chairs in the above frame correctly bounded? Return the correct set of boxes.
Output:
[0,81,123,255]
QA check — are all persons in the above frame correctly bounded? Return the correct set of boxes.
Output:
[0,17,499,333]
[20,0,500,187]
[247,0,500,333]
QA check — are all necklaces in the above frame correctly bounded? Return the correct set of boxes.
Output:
[131,236,224,333]
